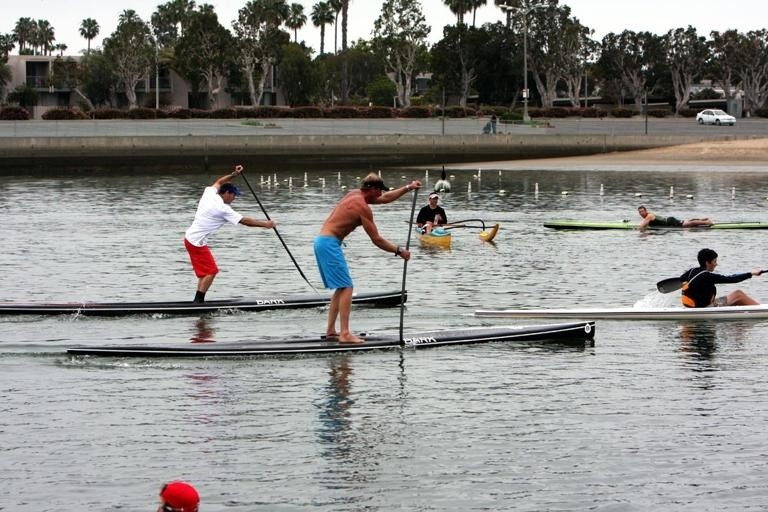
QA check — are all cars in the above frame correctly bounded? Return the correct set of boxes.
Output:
[696,108,736,126]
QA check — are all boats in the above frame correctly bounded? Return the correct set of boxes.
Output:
[413,227,453,252]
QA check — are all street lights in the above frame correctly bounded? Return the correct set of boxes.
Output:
[145,31,170,109]
[499,4,549,120]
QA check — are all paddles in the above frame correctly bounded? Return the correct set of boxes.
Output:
[656,270,766,293]
[238,166,323,299]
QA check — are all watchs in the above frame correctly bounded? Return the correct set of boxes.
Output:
[395,245,404,257]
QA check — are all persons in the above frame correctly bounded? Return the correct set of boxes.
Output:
[415,191,448,235]
[312,171,420,346]
[184,161,276,302]
[679,248,765,307]
[637,202,714,232]
[318,353,357,478]
[677,321,722,422]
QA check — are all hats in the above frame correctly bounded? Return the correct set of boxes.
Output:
[360,180,389,191]
[219,185,246,196]
[429,194,439,199]
